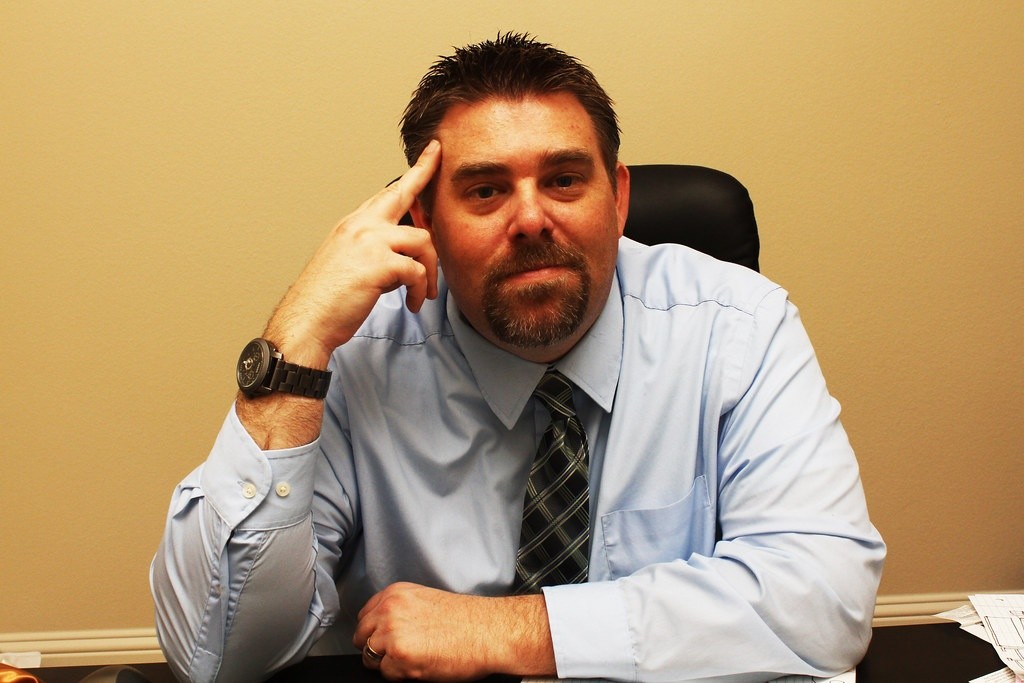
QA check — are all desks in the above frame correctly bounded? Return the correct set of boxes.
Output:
[0,623,1005,683]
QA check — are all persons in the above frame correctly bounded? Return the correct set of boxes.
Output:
[148,29,887,683]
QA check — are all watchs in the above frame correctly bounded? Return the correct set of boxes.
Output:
[235,338,333,399]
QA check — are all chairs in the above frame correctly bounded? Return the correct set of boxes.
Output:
[385,163,759,295]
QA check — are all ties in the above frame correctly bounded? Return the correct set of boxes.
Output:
[510,368,591,595]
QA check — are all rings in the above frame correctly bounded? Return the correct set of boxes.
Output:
[364,638,382,661]
